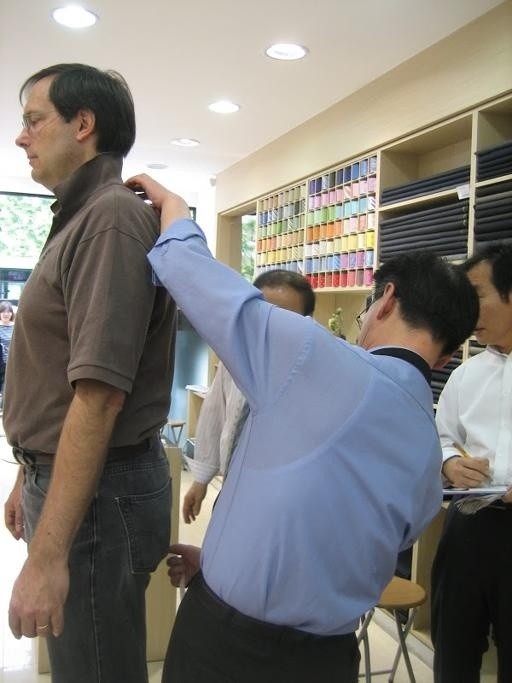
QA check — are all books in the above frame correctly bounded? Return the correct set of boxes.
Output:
[441,485,510,497]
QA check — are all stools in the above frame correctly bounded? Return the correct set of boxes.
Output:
[356,576,427,683]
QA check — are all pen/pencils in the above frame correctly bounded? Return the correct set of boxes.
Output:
[452,441,492,481]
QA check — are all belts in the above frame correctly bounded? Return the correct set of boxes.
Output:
[20,438,165,468]
[185,571,355,648]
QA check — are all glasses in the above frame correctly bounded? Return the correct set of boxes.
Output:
[20,109,58,129]
[356,291,384,329]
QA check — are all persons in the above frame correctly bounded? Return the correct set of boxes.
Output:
[181,270,317,529]
[1,300,14,409]
[0,62,179,683]
[122,172,482,683]
[434,239,512,683]
